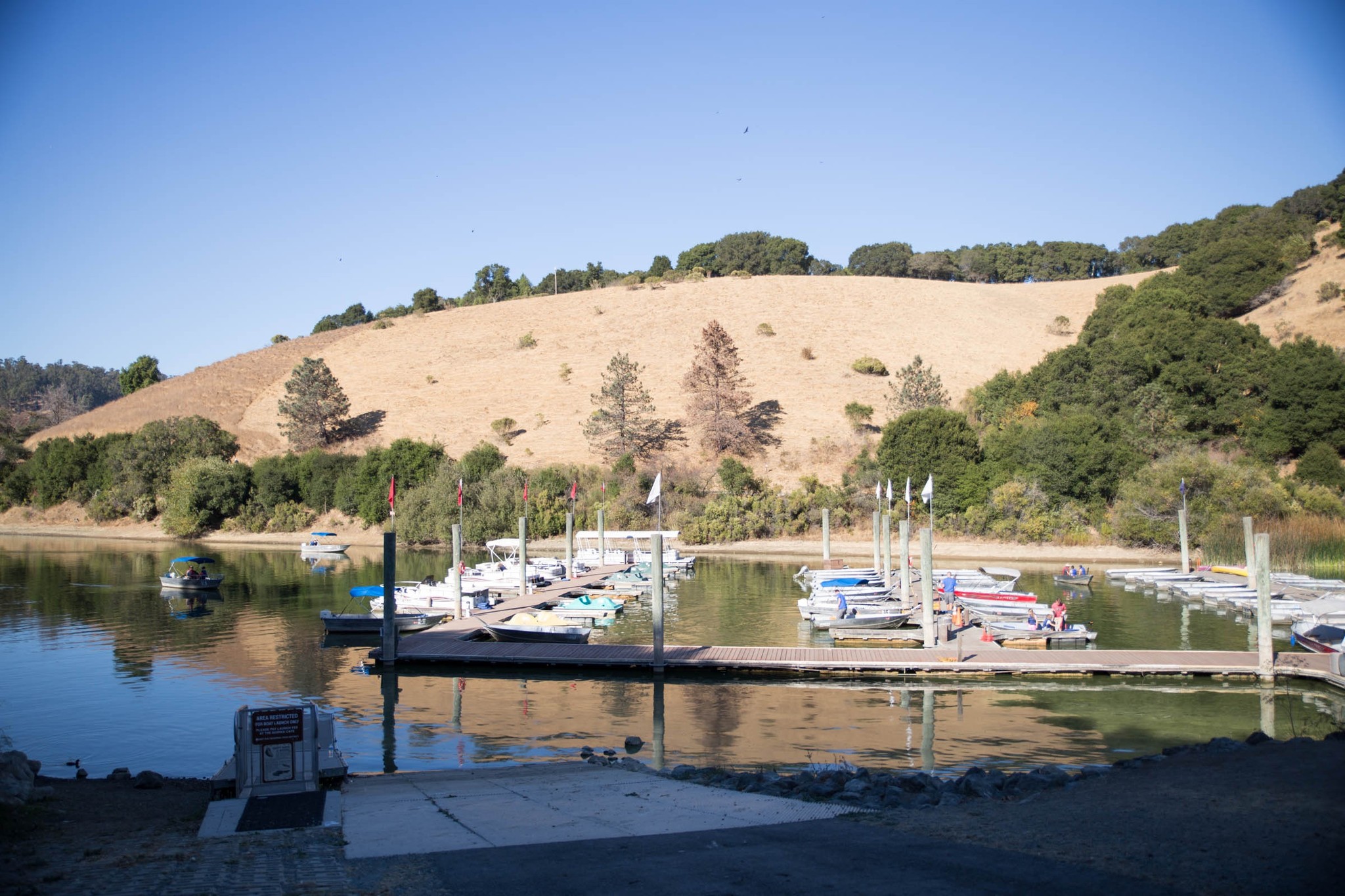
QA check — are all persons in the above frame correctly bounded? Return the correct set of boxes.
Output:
[835,590,847,619]
[1026,598,1068,632]
[309,539,320,546]
[185,565,209,579]
[1062,565,1086,578]
[941,572,957,616]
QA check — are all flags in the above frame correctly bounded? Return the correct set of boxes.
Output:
[458,480,464,506]
[601,481,606,494]
[905,477,910,504]
[885,479,893,501]
[523,482,528,502]
[922,475,933,504]
[387,475,396,511]
[571,482,577,499]
[876,481,881,500]
[646,473,661,505]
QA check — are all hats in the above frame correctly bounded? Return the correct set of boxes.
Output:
[190,566,193,568]
[1065,564,1070,566]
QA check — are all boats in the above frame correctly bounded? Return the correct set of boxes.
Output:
[160,587,224,619]
[159,556,225,589]
[301,532,350,552]
[320,585,448,631]
[483,530,696,644]
[300,552,350,585]
[911,566,1098,641]
[369,539,590,615]
[797,568,913,629]
[1052,574,1094,585]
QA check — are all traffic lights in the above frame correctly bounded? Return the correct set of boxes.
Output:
[1105,566,1345,654]
